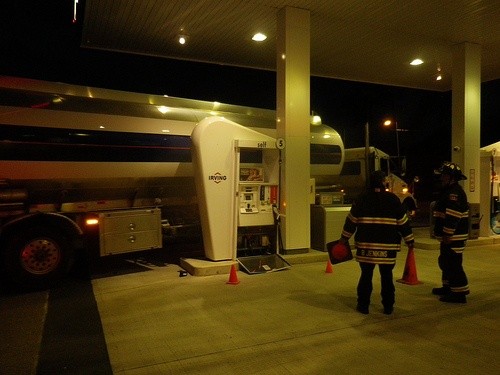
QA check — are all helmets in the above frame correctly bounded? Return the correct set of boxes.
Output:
[371,171,386,187]
[438,163,462,177]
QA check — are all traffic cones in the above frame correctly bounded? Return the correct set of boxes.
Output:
[396,245,421,286]
[226,265,241,284]
[326,259,333,274]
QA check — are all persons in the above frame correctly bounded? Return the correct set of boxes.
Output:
[430,160,471,305]
[340,171,415,316]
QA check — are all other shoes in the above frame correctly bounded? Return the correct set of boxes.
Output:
[357,305,369,313]
[432,285,452,295]
[384,302,394,313]
[440,294,466,303]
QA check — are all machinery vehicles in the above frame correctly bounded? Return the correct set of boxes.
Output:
[1,76,419,292]
